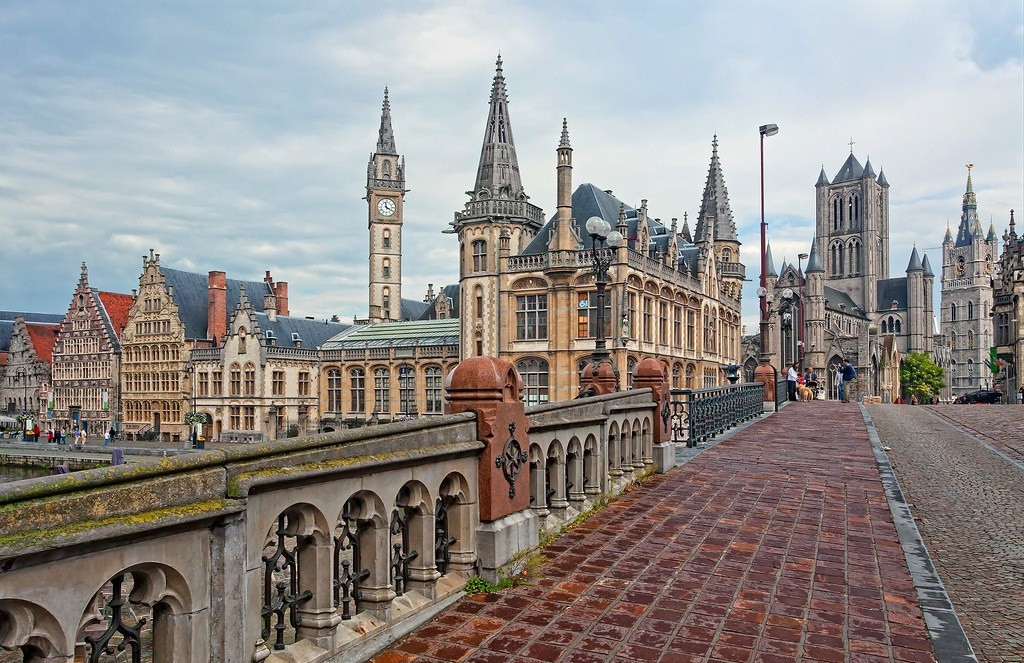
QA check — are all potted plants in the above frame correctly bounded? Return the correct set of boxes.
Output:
[4,431,10,439]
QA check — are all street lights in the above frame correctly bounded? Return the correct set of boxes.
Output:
[585,216,626,363]
[14,366,27,440]
[182,361,196,448]
[781,287,794,367]
[796,252,809,374]
[757,124,780,365]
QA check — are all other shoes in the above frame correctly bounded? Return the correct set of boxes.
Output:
[841,400,847,403]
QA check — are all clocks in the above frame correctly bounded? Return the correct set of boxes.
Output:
[377,198,396,217]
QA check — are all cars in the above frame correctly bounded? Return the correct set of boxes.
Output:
[953,391,995,404]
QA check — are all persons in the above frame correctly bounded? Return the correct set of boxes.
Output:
[787,361,799,401]
[834,362,844,401]
[1017,382,1024,404]
[404,415,414,422]
[104,426,116,446]
[47,426,66,444]
[74,428,86,445]
[911,392,957,405]
[796,373,805,400]
[837,358,856,403]
[804,367,817,388]
[33,423,41,442]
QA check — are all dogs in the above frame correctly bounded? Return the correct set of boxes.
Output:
[798,384,813,402]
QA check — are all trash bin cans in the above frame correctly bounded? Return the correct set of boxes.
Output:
[25,431,35,442]
[197,437,206,449]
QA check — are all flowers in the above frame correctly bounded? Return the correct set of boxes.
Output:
[15,410,37,422]
[183,409,208,427]
